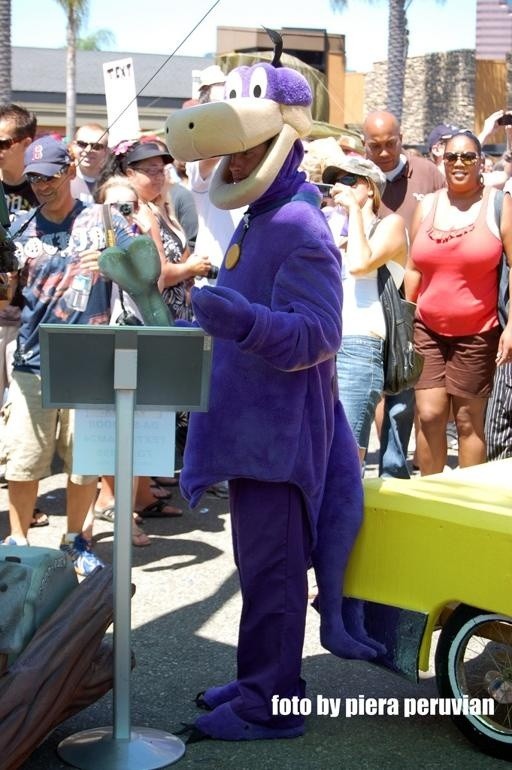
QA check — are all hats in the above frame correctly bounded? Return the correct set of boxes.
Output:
[197,64,227,92]
[322,156,387,198]
[121,143,174,172]
[20,135,72,179]
[427,124,472,151]
[339,135,366,154]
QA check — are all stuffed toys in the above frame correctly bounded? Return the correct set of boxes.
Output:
[164,61,380,743]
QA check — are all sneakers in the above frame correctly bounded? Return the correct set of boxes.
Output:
[0,536,31,547]
[59,531,106,577]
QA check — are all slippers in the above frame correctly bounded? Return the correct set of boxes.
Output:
[30,474,183,547]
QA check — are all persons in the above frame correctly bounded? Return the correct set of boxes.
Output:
[0,101,512,556]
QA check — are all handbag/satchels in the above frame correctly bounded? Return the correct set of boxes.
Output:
[370,281,425,396]
[107,279,143,327]
[497,252,510,330]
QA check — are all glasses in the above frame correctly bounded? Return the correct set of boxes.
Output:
[330,173,358,187]
[442,150,478,167]
[25,173,52,185]
[77,140,104,150]
[133,168,168,176]
[0,134,21,150]
[119,203,132,216]
